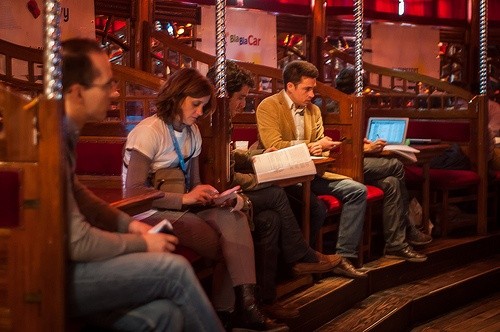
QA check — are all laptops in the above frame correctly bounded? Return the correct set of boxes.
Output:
[365,118,410,145]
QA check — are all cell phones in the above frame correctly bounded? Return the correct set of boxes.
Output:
[339,137,346,142]
[218,186,241,199]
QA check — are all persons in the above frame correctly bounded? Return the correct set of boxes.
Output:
[327,68,432,262]
[122,68,288,332]
[34,39,227,332]
[206,60,341,318]
[249,60,371,279]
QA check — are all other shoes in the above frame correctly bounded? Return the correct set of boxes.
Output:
[407,228,432,245]
[257,301,299,319]
[384,245,428,262]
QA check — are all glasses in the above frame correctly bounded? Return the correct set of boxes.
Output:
[90,77,120,90]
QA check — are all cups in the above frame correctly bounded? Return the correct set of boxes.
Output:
[236,140,249,151]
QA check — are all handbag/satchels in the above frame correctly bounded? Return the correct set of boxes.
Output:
[152,167,186,193]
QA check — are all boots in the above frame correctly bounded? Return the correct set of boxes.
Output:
[216,311,233,332]
[232,284,289,332]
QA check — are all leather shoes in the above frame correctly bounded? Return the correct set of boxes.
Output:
[292,250,342,274]
[331,257,368,279]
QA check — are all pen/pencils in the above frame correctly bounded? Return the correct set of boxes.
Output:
[376,134,385,147]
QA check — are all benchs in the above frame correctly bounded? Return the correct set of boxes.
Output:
[0,82,488,332]
